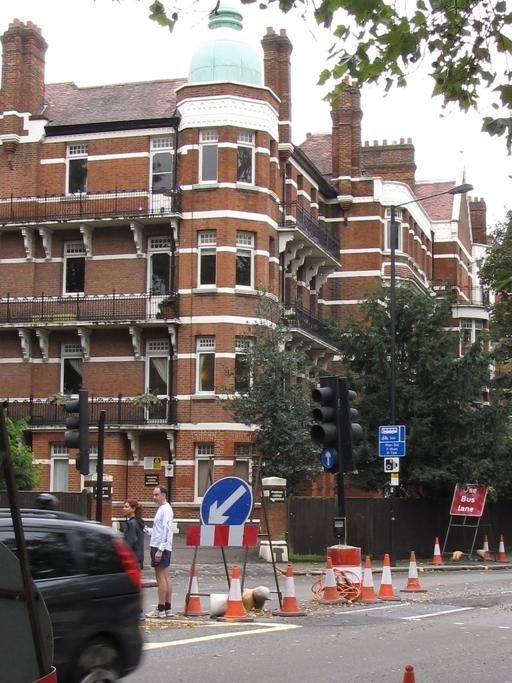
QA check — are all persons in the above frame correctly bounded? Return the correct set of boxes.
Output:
[38,491,61,511]
[120,499,147,574]
[144,488,174,617]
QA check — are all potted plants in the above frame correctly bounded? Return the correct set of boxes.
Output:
[158,295,177,319]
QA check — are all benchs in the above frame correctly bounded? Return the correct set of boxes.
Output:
[31,313,77,321]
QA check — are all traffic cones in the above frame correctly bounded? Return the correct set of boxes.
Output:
[316,554,344,605]
[357,554,384,604]
[375,552,401,602]
[176,561,208,617]
[216,560,255,621]
[428,536,447,566]
[272,561,309,617]
[479,533,493,561]
[401,665,416,682]
[398,549,428,594]
[497,533,507,560]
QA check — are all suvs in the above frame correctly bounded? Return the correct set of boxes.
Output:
[0,503,144,683]
[0,541,60,683]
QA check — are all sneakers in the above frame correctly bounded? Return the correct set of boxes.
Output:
[137,611,145,621]
[145,607,174,618]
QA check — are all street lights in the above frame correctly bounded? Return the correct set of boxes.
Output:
[386,183,475,569]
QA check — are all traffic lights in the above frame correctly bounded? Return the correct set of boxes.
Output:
[309,373,346,475]
[337,374,365,473]
[62,387,91,475]
